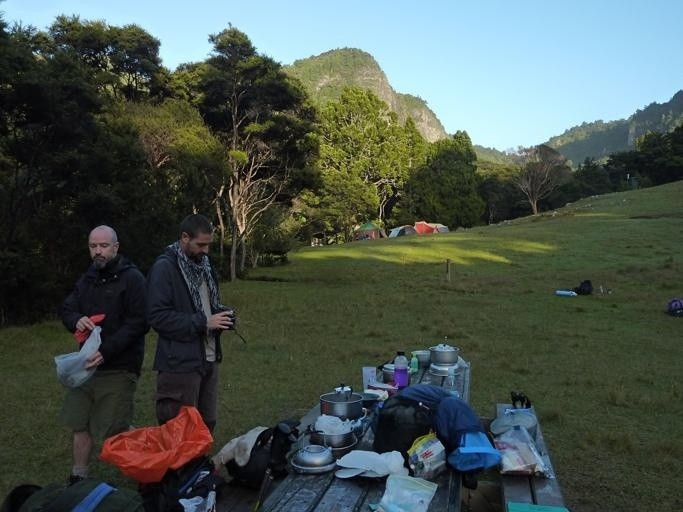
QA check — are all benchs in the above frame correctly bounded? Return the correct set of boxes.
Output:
[495,401,568,511]
[210,407,311,512]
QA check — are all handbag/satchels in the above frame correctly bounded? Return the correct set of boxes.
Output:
[165,459,227,512]
[1,481,145,511]
[226,420,300,487]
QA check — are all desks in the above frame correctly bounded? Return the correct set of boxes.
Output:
[256,362,469,512]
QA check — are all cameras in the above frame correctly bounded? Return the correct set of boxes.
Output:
[218,304,237,330]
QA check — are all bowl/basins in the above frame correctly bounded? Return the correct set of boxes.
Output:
[413,349,431,363]
[367,383,397,398]
[430,346,460,377]
[359,392,379,407]
[310,419,354,448]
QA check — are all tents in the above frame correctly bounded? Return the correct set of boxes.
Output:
[354,221,450,240]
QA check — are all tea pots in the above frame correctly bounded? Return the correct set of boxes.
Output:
[290,430,338,466]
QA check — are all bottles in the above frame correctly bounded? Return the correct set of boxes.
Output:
[393,350,409,389]
[411,352,419,373]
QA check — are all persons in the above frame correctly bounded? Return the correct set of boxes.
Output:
[59,221,150,490]
[145,212,235,485]
